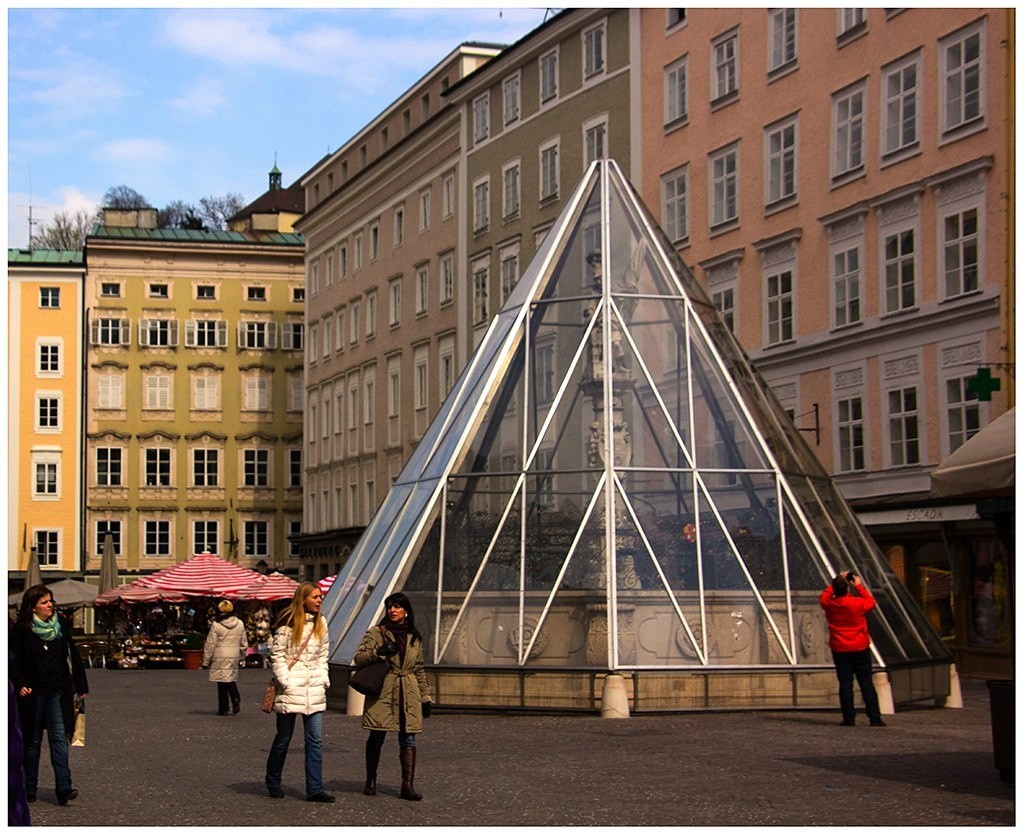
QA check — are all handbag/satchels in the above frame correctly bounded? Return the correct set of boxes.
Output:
[70,696,86,749]
[261,677,279,714]
[348,622,393,697]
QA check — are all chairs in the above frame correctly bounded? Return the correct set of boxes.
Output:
[93,641,112,669]
[76,642,95,668]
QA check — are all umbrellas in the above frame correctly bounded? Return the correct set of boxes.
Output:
[98,520,118,597]
[22,546,44,592]
[8,576,100,619]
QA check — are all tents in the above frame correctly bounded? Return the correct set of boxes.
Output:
[93,546,367,638]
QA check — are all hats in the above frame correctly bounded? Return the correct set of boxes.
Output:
[217,599,234,613]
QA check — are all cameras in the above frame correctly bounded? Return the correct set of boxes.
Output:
[845,572,856,582]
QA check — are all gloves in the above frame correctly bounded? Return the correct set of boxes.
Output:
[420,699,432,718]
[376,640,399,657]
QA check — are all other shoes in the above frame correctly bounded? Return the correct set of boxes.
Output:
[27,790,37,803]
[233,698,241,715]
[59,787,81,806]
[870,717,885,727]
[841,717,857,726]
[266,784,284,799]
[217,709,230,716]
[308,791,336,803]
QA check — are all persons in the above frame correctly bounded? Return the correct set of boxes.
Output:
[200,599,248,716]
[265,582,338,803]
[8,583,90,826]
[819,570,887,727]
[352,592,432,800]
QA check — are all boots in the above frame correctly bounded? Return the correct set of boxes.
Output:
[398,745,423,800]
[363,744,383,796]
